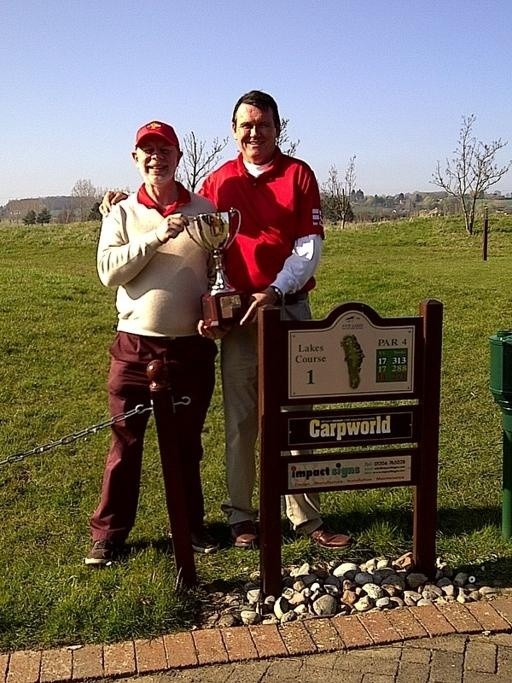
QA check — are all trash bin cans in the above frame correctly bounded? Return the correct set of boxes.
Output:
[272,287,282,299]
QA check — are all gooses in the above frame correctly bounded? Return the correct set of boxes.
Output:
[181,207,252,330]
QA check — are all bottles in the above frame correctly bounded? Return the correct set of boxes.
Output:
[134,121,179,150]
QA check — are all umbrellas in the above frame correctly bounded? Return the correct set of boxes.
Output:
[229,520,258,548]
[307,525,352,550]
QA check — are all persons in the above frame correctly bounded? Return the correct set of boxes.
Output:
[85,121,230,565]
[99,90,353,550]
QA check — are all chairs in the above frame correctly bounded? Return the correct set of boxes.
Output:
[85,540,122,566]
[168,530,218,554]
